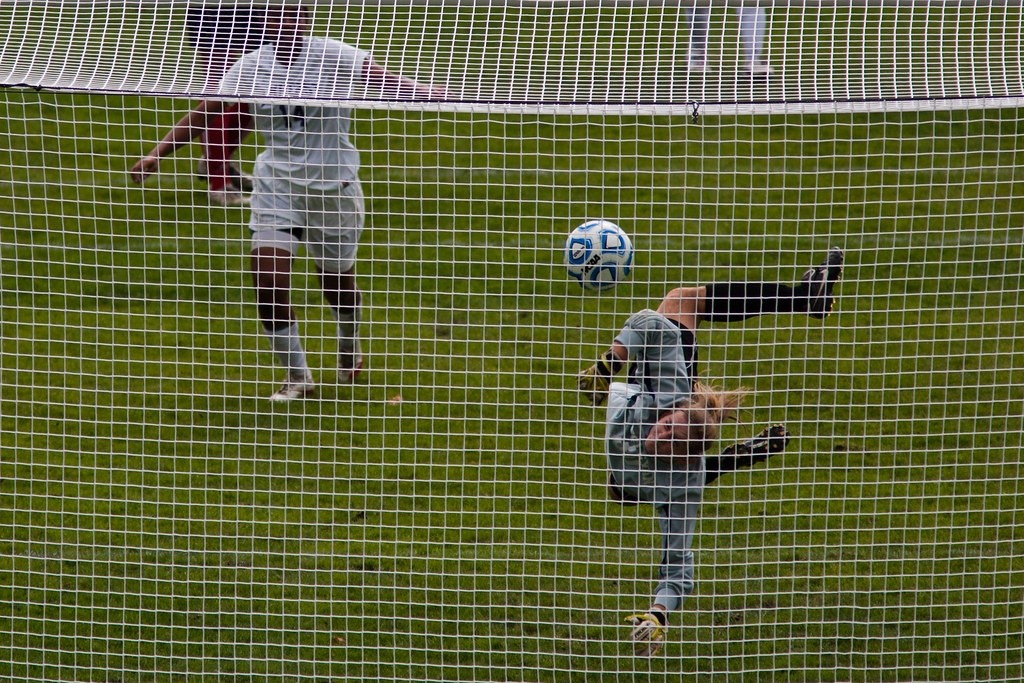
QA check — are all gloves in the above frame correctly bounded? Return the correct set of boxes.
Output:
[624,608,665,659]
[575,348,623,408]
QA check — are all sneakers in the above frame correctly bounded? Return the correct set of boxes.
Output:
[270,372,316,403]
[719,423,793,473]
[800,246,843,321]
[335,319,363,382]
[207,185,250,207]
[197,153,255,191]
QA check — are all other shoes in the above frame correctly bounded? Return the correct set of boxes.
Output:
[743,64,775,81]
[687,47,714,76]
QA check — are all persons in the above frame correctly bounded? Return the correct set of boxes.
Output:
[130,5,450,403]
[575,246,847,661]
[187,0,266,204]
[681,0,776,81]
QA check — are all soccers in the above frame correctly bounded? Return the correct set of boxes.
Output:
[563,220,635,292]
